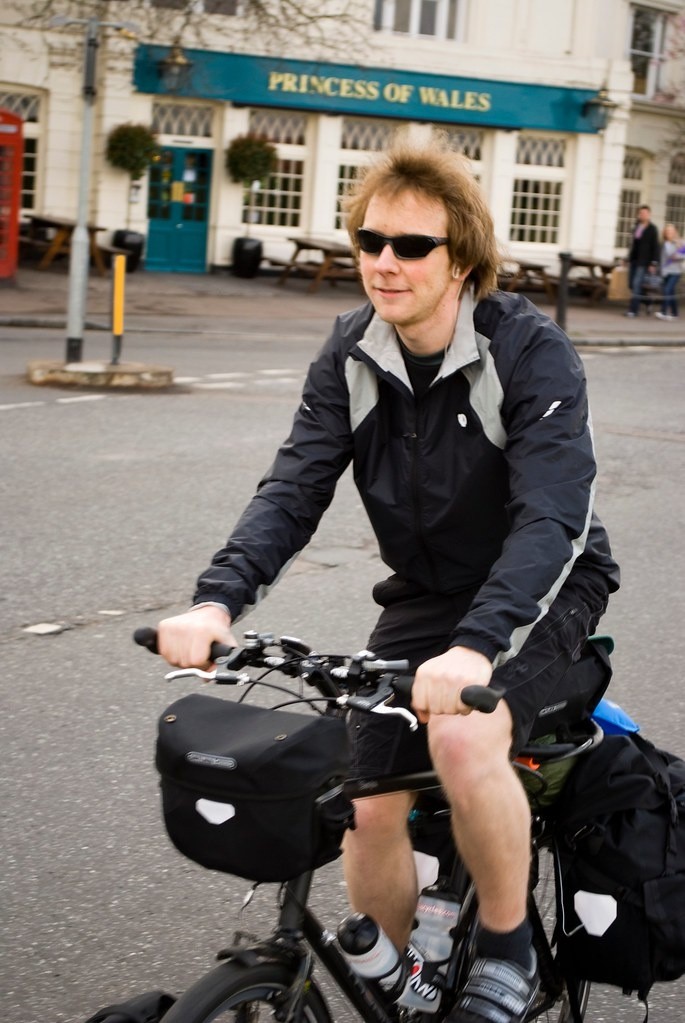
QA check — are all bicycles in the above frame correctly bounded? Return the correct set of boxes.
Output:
[130,621,608,1022]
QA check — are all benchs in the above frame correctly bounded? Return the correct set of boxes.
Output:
[16,237,131,276]
[494,274,658,308]
[262,258,367,296]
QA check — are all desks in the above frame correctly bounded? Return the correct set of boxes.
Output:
[568,256,621,305]
[24,214,106,278]
[278,238,357,294]
[499,254,558,304]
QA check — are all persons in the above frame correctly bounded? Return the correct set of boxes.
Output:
[622,205,685,319]
[157,152,620,1023]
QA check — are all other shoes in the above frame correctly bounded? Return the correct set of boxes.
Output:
[655,311,664,319]
[623,312,638,318]
[664,315,677,322]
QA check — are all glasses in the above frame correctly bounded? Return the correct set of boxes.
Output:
[356,227,450,260]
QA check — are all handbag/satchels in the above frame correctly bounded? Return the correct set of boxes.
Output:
[641,273,662,292]
[547,733,685,1002]
[607,265,632,299]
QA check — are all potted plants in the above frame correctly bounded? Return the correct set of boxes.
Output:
[226,134,277,278]
[102,124,160,272]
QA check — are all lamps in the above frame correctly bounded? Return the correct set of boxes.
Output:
[156,43,191,92]
[577,88,618,133]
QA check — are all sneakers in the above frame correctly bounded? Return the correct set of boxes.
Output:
[443,944,541,1023]
